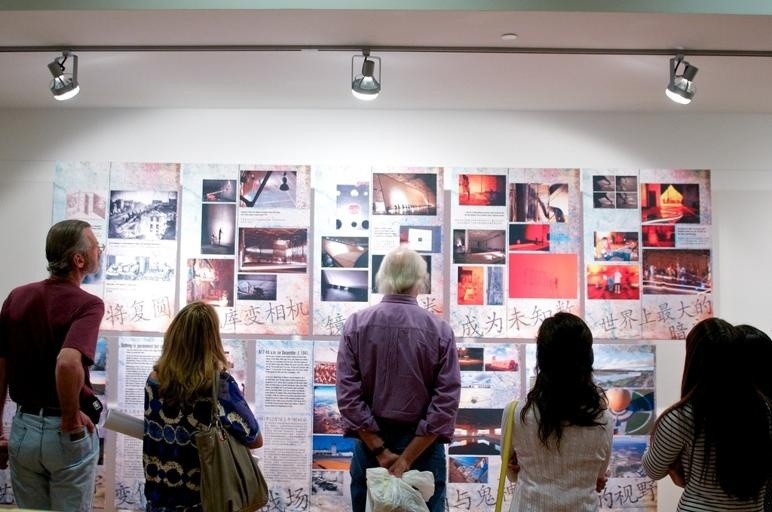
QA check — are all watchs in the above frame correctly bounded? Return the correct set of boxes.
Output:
[371,442,388,456]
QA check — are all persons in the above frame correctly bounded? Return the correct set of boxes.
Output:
[500,312,616,512]
[600,269,623,295]
[142,301,264,512]
[641,261,709,288]
[642,318,771,512]
[336,247,461,512]
[0,220,105,512]
[734,325,772,400]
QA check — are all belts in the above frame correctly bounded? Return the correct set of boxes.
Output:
[19,403,62,418]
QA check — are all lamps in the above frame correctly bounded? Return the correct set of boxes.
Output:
[348,52,382,101]
[664,54,700,106]
[47,54,81,102]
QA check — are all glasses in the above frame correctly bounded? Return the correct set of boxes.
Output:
[87,242,108,251]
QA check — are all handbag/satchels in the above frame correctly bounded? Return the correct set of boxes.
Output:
[194,369,269,512]
